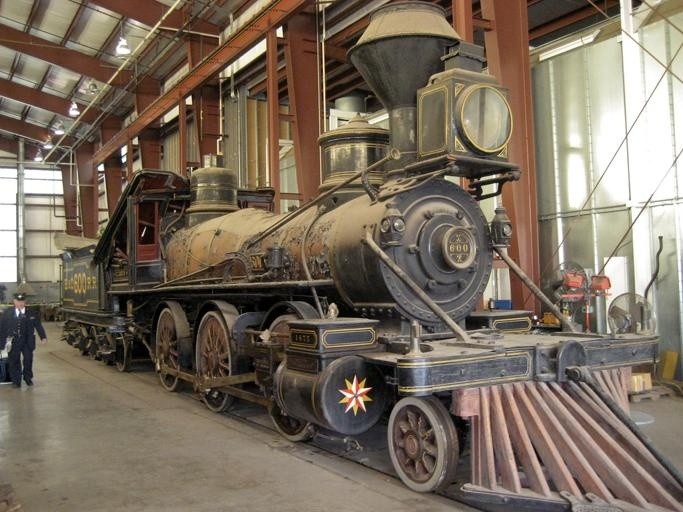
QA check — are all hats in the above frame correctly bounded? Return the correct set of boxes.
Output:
[11,292,26,301]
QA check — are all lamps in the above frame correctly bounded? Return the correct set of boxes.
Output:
[34,117,65,162]
[116,20,130,54]
[68,89,80,115]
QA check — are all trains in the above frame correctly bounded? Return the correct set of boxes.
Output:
[54,0,682,510]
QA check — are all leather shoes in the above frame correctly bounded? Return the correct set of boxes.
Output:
[11,377,32,388]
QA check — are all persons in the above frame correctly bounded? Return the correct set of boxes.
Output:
[0,293,47,389]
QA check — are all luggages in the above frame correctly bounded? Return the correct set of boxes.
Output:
[0,348,13,382]
